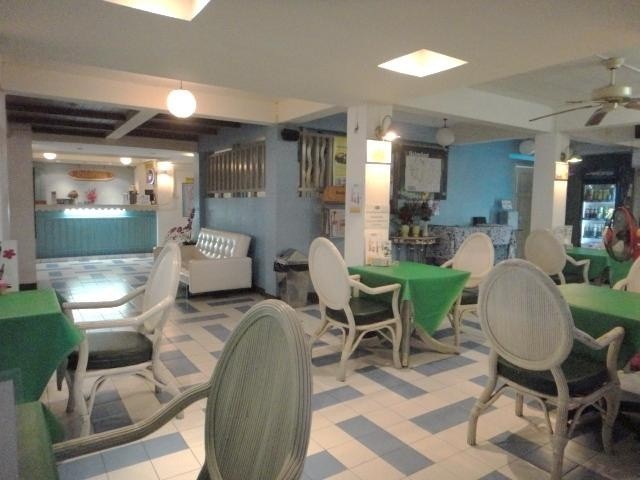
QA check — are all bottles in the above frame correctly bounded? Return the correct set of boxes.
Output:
[585,186,613,238]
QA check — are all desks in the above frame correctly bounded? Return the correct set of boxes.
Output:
[1,286,83,404]
[392,236,441,264]
[516,284,640,417]
[348,260,471,353]
[562,246,627,289]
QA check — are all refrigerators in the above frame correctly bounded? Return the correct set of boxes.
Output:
[578,166,635,253]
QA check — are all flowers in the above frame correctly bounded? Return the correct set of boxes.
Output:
[400,201,431,222]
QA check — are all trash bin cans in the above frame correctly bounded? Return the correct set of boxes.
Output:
[274,249,309,309]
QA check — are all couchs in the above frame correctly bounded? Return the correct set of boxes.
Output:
[156,228,253,300]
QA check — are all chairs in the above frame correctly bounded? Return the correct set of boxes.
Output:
[441,232,495,347]
[54,297,315,480]
[525,230,589,283]
[62,242,181,439]
[466,255,625,478]
[309,236,403,383]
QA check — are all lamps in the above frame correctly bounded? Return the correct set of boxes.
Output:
[560,143,583,163]
[436,117,455,147]
[374,113,401,141]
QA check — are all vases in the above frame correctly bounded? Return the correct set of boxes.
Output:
[402,220,428,237]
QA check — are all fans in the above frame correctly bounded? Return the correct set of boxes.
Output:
[527,57,640,129]
